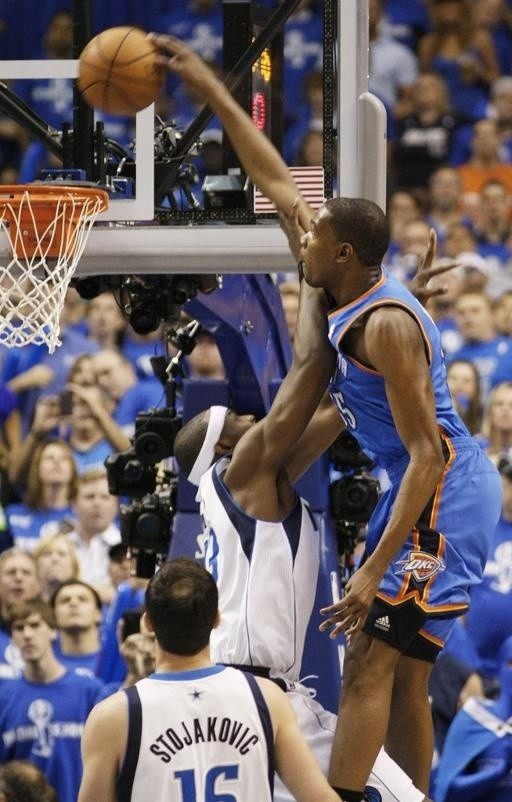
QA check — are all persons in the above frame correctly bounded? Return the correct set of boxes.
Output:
[1,1,510,802]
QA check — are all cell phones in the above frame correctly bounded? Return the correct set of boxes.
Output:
[58,391,74,415]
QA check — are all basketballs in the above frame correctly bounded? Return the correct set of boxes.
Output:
[79,28,164,119]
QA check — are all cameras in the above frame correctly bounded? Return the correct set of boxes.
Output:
[324,429,376,470]
[133,406,182,464]
[119,275,187,336]
[119,502,173,553]
[331,478,382,523]
[103,446,159,500]
